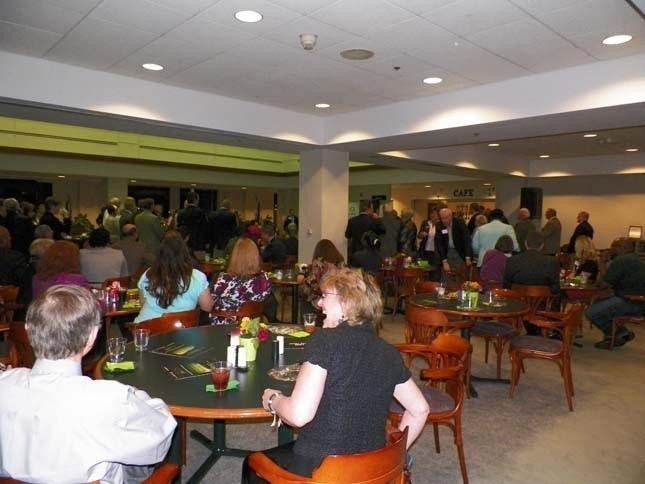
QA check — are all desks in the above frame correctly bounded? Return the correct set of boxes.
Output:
[562,280,611,338]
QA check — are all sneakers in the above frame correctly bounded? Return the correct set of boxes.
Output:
[595,325,635,349]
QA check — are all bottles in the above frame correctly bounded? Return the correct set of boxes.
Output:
[104,286,120,303]
[275,267,294,280]
[225,345,247,370]
[271,334,285,360]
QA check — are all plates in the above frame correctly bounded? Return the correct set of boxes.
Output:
[266,363,304,382]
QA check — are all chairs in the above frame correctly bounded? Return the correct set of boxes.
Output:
[392,266,586,412]
[609,295,645,351]
[393,344,469,484]
[0,256,408,483]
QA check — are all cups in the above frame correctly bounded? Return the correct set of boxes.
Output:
[210,362,232,392]
[435,281,497,311]
[108,327,151,360]
[303,312,317,332]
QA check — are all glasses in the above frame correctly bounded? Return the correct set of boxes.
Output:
[322,291,341,298]
